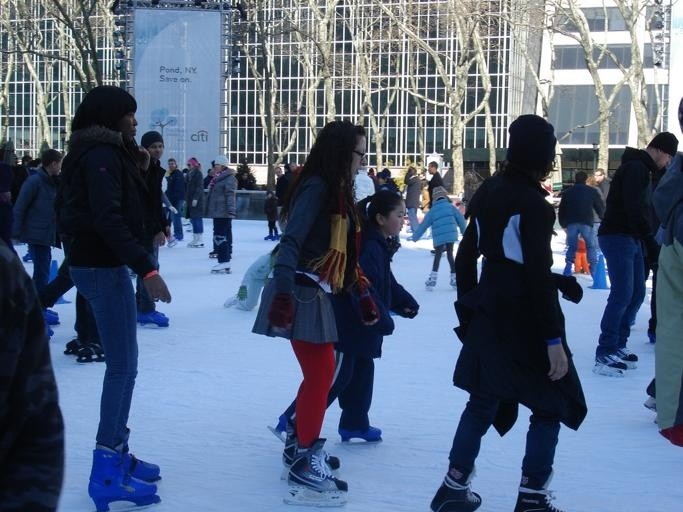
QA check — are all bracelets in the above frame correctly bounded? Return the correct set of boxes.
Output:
[145,270,158,280]
[542,338,563,345]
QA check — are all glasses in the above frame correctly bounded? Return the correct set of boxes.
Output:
[352,148,368,162]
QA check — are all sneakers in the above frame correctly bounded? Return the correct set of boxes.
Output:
[136,311,170,324]
[339,425,382,441]
[66,337,103,356]
[614,347,638,362]
[279,414,291,426]
[264,234,280,240]
[209,250,231,271]
[595,353,627,370]
[42,309,59,322]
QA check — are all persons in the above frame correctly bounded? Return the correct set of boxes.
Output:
[1,136,105,363]
[250,120,380,493]
[266,192,420,444]
[127,132,305,328]
[429,114,588,511]
[541,132,682,448]
[351,162,484,288]
[55,86,171,503]
[0,233,66,511]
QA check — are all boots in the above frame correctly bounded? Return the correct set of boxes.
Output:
[188,232,205,246]
[424,271,438,287]
[514,468,567,512]
[88,428,160,497]
[430,465,482,512]
[450,273,457,286]
[279,419,349,493]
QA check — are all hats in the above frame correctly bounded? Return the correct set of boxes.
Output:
[141,130,164,150]
[214,155,228,167]
[506,114,556,171]
[650,132,679,158]
[432,185,452,202]
[187,158,199,166]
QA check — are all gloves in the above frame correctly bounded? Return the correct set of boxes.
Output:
[360,295,381,325]
[143,275,172,304]
[192,200,197,206]
[660,423,682,445]
[269,294,293,330]
[154,227,171,246]
[168,205,177,212]
